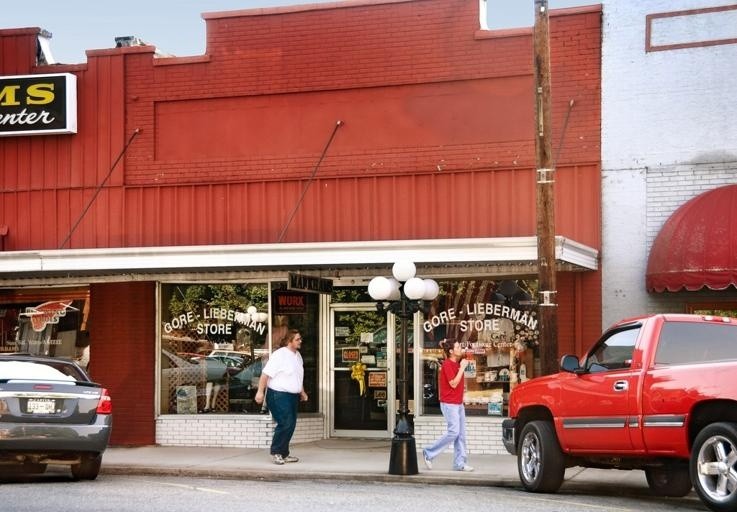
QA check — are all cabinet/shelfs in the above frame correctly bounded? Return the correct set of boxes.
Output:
[460,345,534,416]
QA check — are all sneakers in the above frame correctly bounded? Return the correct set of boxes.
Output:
[423,450,432,470]
[453,464,474,471]
[271,453,298,465]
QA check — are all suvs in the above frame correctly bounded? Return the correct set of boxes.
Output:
[357,320,533,407]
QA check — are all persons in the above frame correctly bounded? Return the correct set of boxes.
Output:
[253,329,309,466]
[202,344,230,413]
[420,336,474,472]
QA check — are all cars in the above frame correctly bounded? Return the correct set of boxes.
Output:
[0,355,113,482]
[160,348,269,414]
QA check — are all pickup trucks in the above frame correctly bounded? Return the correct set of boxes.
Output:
[502,313,737,512]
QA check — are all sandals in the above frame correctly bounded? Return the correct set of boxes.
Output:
[199,408,216,413]
[259,406,267,414]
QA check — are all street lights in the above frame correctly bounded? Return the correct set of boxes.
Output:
[368,262,440,476]
[239,306,269,362]
